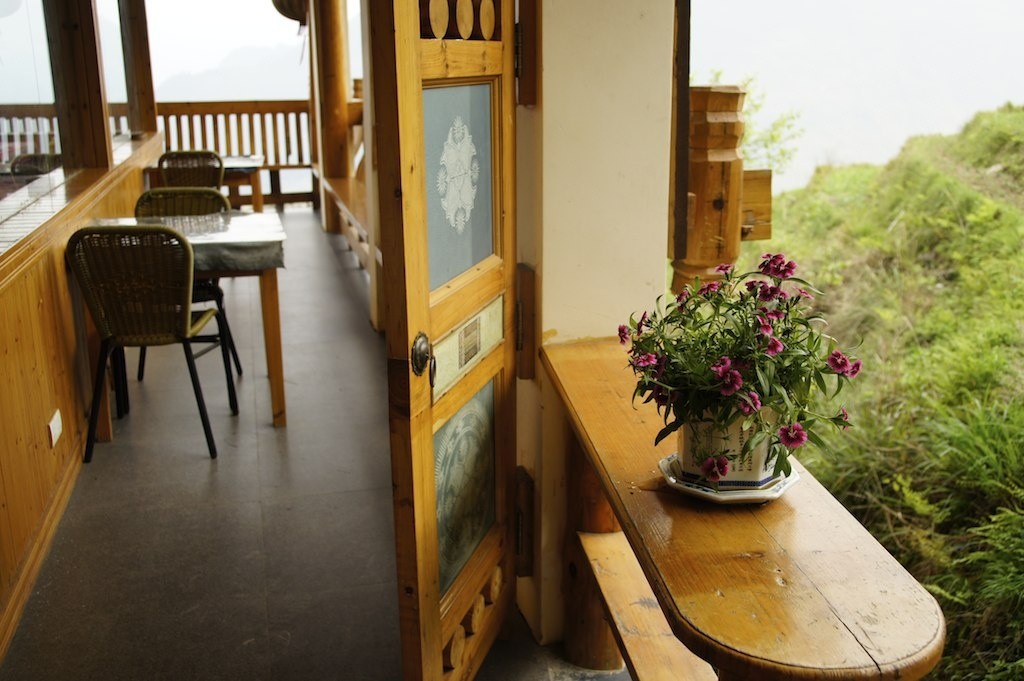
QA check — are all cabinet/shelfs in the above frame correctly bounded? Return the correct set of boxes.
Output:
[541,330,947,680]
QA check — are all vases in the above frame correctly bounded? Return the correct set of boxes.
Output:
[657,402,792,503]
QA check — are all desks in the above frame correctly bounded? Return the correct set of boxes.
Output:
[88,212,289,428]
[151,154,265,212]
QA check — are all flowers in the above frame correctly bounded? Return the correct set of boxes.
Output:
[613,250,865,491]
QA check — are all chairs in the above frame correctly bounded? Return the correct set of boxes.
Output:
[11,153,62,176]
[132,186,244,372]
[65,224,240,460]
[158,150,225,188]
